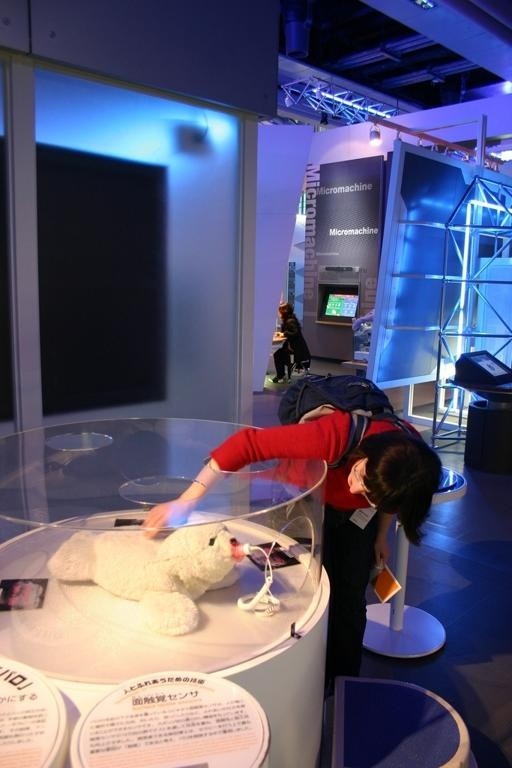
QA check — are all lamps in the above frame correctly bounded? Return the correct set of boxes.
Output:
[281,76,503,174]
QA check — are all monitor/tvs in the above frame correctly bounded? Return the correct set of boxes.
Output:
[319,288,358,322]
[469,353,512,377]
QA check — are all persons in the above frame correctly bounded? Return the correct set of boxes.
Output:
[143,371,444,699]
[272,301,310,382]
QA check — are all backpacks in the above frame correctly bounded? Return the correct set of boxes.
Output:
[277,372,417,472]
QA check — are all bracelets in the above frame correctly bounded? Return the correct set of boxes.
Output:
[191,479,208,489]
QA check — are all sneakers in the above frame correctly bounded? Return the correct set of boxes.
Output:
[272,373,286,384]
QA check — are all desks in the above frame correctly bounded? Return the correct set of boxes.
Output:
[272,332,287,347]
[361,466,469,659]
